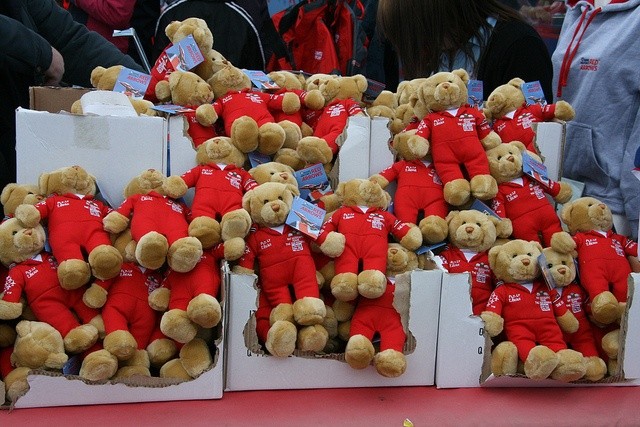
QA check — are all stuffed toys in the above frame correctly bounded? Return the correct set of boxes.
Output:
[196,66,301,154]
[395,77,428,125]
[550,196,638,324]
[103,168,203,273]
[11,320,69,369]
[0,322,33,405]
[143,15,214,103]
[333,241,418,378]
[1,182,45,224]
[249,162,298,188]
[408,68,504,205]
[368,122,448,249]
[155,70,219,147]
[303,72,338,128]
[82,227,164,364]
[16,164,123,289]
[231,180,346,325]
[296,73,369,164]
[0,216,105,353]
[437,209,502,316]
[482,77,576,155]
[161,136,260,247]
[486,140,574,246]
[266,70,325,148]
[366,90,403,133]
[479,238,588,382]
[315,177,422,300]
[541,246,619,381]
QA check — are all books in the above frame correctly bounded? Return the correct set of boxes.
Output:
[519,80,547,106]
[243,69,281,90]
[285,195,327,239]
[470,198,503,220]
[291,161,334,204]
[415,242,447,255]
[112,67,153,100]
[164,33,205,71]
[521,150,549,188]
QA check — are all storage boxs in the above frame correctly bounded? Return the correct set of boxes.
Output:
[223,251,441,392]
[370,115,567,214]
[330,117,370,193]
[435,256,639,389]
[16,87,198,209]
[1,258,227,409]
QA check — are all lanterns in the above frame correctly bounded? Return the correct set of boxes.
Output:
[148,236,245,343]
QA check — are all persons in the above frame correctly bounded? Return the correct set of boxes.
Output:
[543,1,635,225]
[370,0,553,106]
[0,0,151,86]
[75,0,136,54]
